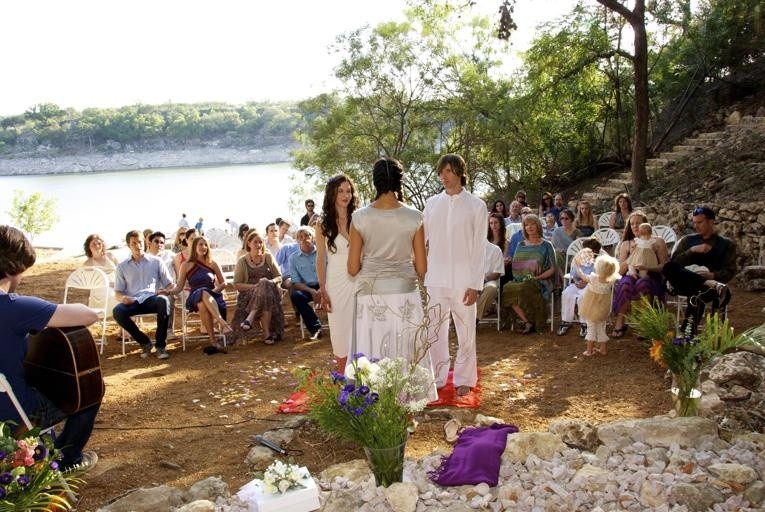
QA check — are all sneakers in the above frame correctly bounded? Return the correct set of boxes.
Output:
[579,324,587,337]
[557,322,573,336]
[156,347,169,358]
[80,451,98,472]
[140,342,154,358]
[306,327,322,340]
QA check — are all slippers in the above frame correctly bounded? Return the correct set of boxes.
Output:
[264,335,275,346]
[240,320,252,330]
[221,323,233,335]
[611,326,625,337]
[444,417,461,442]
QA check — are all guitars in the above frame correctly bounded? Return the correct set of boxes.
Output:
[22,327,102,415]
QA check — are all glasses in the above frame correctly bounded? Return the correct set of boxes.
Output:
[518,195,525,198]
[542,197,551,201]
[307,205,314,207]
[693,208,704,214]
[561,216,569,220]
[154,239,165,244]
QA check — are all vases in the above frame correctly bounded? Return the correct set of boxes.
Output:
[362,444,405,486]
[671,373,700,417]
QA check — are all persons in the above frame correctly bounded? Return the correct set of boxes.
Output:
[83,196,321,359]
[450,191,738,356]
[314,174,364,376]
[341,157,438,435]
[0,223,102,476]
[423,154,489,397]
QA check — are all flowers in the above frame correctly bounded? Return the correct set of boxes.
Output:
[0,420,88,511]
[288,352,437,487]
[617,292,765,417]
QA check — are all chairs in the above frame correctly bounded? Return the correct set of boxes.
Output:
[0,372,78,511]
[63,226,330,356]
[475,211,736,339]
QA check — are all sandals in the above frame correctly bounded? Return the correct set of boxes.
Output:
[712,281,728,297]
[520,321,537,335]
